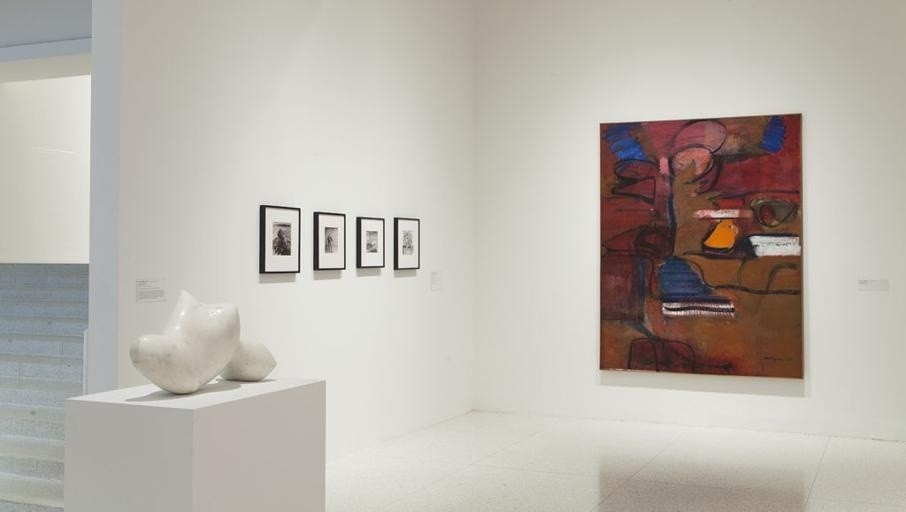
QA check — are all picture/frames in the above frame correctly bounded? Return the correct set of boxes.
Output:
[257,203,421,275]
